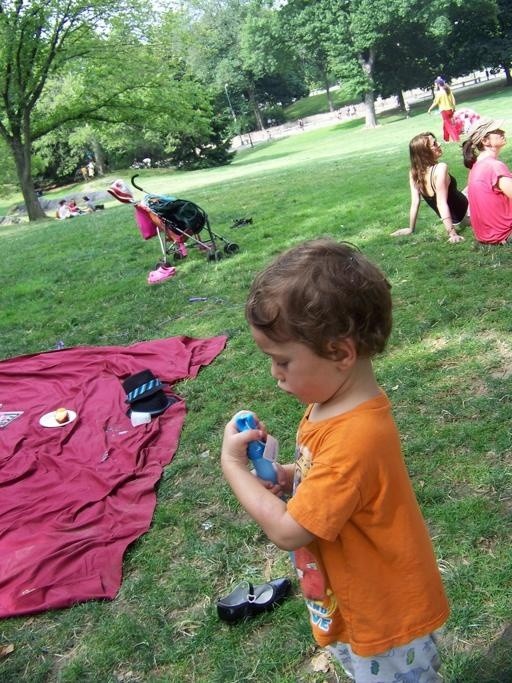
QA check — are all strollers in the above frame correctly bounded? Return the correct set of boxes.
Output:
[128,174,238,270]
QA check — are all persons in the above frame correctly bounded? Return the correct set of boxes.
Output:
[82,159,94,182]
[219,235,452,682]
[404,101,411,118]
[58,196,95,219]
[390,131,470,245]
[428,76,460,142]
[459,117,512,244]
[131,157,169,170]
[338,105,358,120]
[297,115,304,130]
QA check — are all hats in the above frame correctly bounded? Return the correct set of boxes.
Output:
[466,116,503,144]
[121,369,175,419]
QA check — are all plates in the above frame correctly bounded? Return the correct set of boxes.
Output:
[38,407,78,427]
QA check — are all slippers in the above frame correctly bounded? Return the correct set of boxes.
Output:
[148,264,176,284]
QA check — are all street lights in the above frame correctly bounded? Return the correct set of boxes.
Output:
[224,83,244,145]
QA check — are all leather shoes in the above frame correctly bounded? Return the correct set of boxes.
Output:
[216,578,291,623]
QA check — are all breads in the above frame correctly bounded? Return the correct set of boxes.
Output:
[55,408,69,423]
